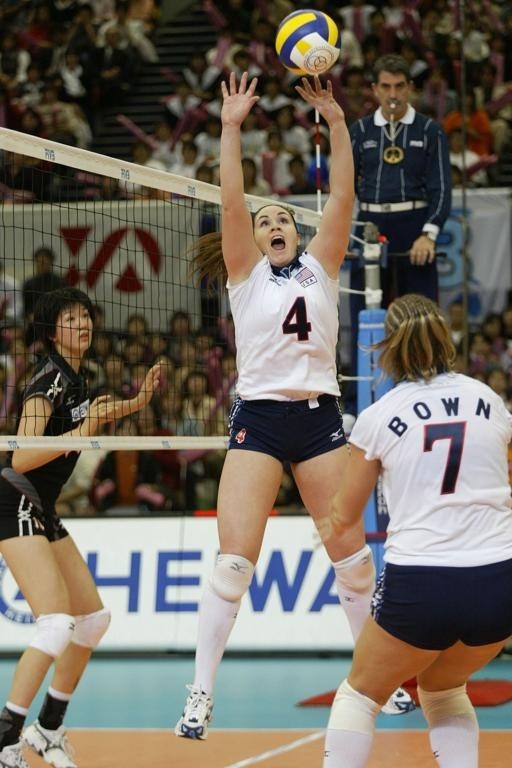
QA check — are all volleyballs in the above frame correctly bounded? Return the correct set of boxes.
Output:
[275,9,340,76]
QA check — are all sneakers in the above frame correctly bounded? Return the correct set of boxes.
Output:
[18,718,79,768]
[380,687,417,715]
[0,741,31,768]
[174,683,215,741]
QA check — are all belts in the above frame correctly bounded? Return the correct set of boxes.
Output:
[360,199,429,214]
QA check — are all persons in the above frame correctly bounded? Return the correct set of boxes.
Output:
[0,288,162,768]
[1,0,510,516]
[175,71,415,740]
[324,294,512,768]
